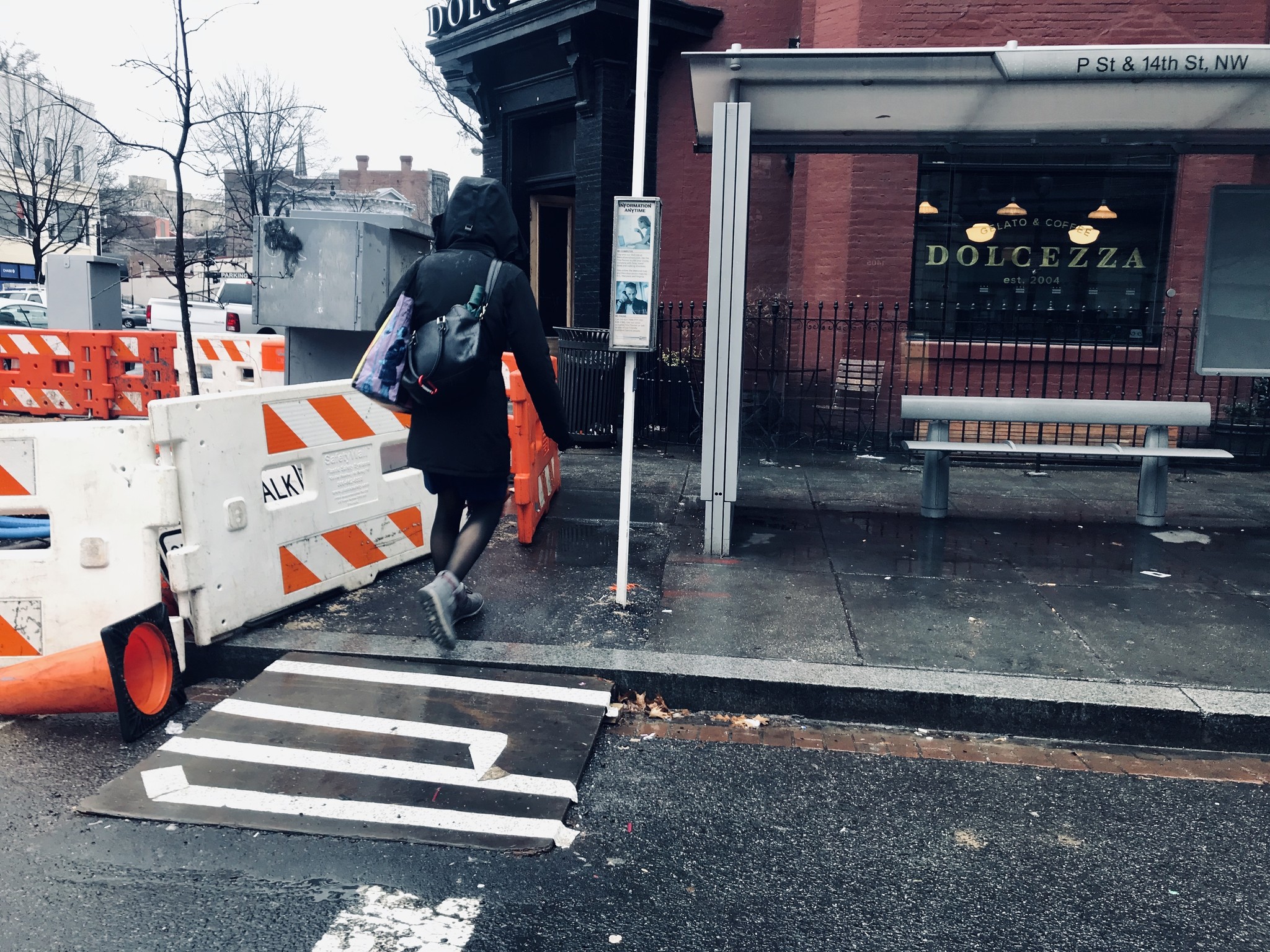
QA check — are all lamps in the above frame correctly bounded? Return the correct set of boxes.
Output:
[919,171,938,214]
[996,172,1027,216]
[1087,185,1117,219]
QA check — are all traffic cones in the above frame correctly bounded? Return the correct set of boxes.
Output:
[3,601,192,745]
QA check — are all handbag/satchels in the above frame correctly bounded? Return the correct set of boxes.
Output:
[351,291,417,414]
[404,259,502,392]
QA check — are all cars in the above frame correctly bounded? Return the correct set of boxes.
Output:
[122,307,148,329]
[0,298,48,330]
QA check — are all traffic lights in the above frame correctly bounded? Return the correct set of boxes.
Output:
[208,252,216,266]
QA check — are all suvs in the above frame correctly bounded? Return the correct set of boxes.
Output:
[0,283,47,306]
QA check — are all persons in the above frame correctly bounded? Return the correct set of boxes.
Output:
[612,282,647,314]
[627,215,653,250]
[375,175,577,647]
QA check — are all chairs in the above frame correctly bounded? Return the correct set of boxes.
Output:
[811,359,886,458]
[685,358,774,461]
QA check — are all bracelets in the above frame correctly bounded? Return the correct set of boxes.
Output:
[619,300,622,304]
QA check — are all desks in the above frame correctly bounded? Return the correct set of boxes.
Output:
[743,366,826,467]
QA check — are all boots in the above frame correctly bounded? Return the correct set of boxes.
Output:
[416,570,460,651]
[451,581,484,626]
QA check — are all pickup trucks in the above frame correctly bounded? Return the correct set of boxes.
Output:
[149,276,286,335]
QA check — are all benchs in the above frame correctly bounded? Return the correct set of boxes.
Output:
[900,395,1234,526]
